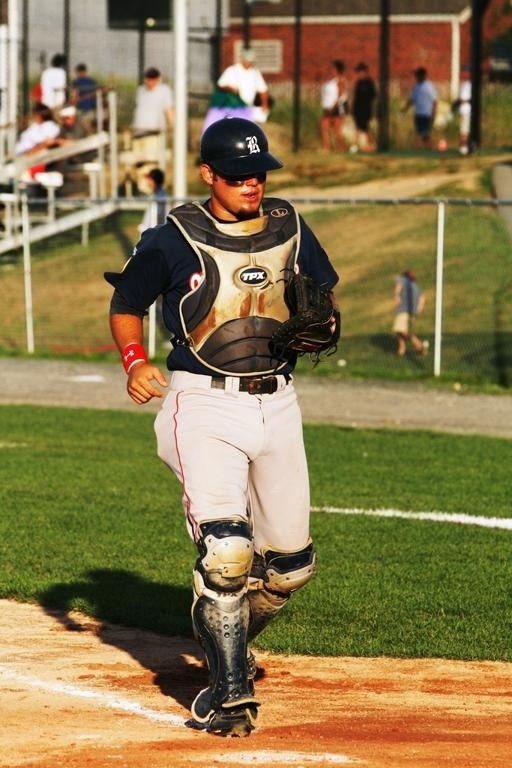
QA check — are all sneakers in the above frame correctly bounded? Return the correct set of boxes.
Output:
[239,645,266,693]
[419,338,431,359]
[189,683,263,741]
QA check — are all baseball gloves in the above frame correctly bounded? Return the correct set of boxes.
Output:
[268,273,338,354]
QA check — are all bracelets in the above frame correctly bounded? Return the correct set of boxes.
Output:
[120,343,147,374]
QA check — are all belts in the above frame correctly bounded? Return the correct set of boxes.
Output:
[171,368,295,394]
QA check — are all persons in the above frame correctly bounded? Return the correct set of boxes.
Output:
[218,50,270,113]
[316,61,471,155]
[110,117,340,734]
[394,272,430,359]
[10,53,172,237]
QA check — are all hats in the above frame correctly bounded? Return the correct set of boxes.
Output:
[199,116,284,178]
[140,66,161,80]
[328,56,472,80]
